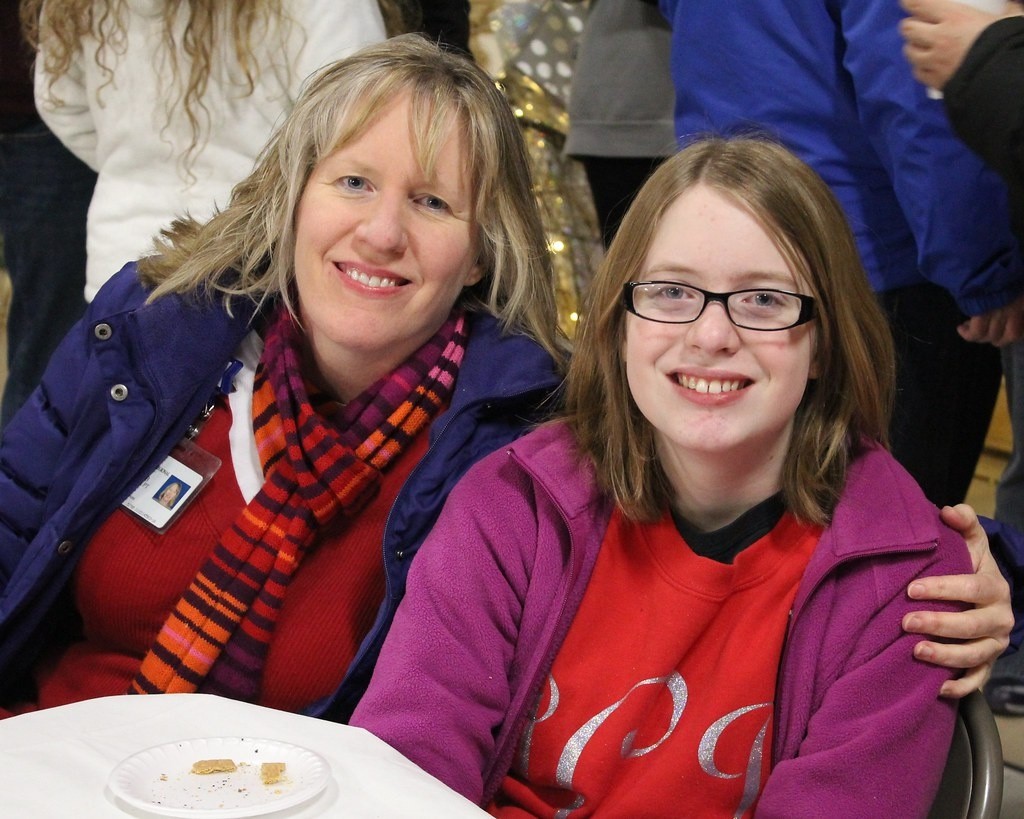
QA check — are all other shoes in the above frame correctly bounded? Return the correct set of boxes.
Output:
[982,674,1024,719]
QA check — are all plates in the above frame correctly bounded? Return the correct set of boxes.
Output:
[107,735,331,818]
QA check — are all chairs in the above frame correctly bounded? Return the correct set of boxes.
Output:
[927,689,1002,819]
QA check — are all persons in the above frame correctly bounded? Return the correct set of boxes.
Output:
[0,0,1024,819]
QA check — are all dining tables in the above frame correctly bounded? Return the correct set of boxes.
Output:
[1,694,497,819]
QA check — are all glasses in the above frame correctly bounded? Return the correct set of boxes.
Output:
[624,281,818,332]
